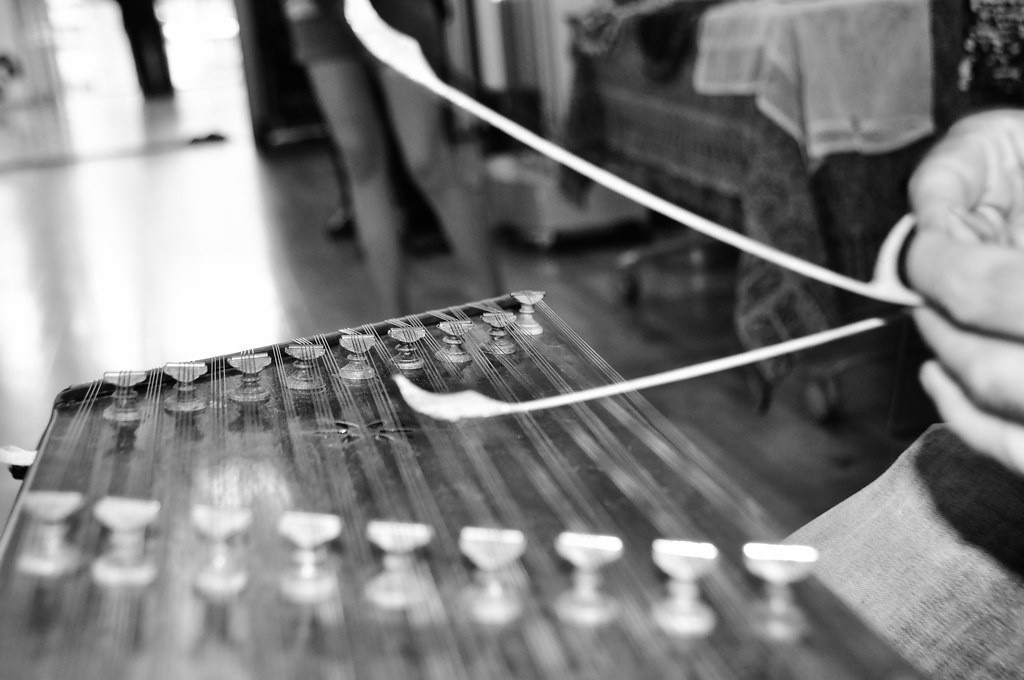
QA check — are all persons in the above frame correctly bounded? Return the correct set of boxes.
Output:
[291,1,505,319]
[784,108,1024,680]
